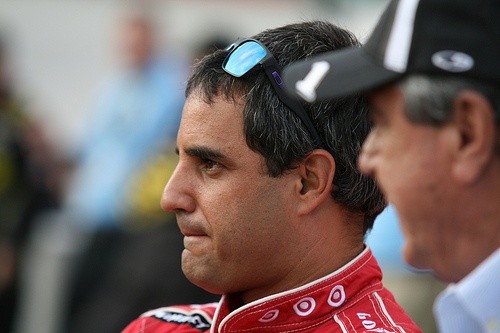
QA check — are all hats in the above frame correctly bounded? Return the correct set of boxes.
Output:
[283,0,500,101]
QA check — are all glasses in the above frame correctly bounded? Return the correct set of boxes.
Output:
[221,39,324,150]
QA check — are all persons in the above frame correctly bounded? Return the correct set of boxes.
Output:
[121,20,424,333]
[283,0,500,333]
